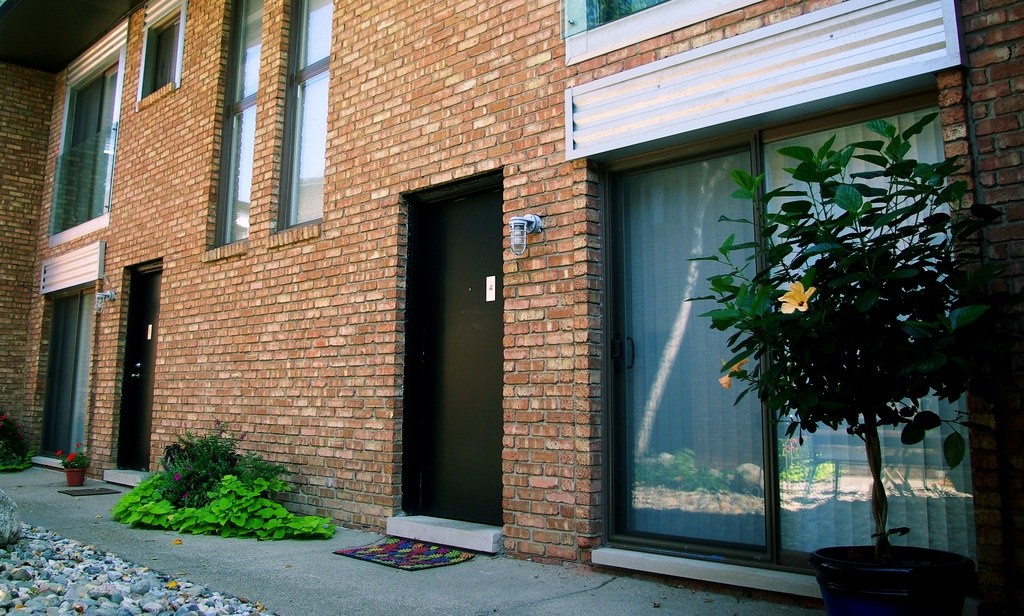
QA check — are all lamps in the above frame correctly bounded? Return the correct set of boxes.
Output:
[508,214,542,256]
[95,290,116,314]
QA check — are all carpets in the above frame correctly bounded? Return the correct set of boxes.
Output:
[57,488,122,496]
[332,535,476,571]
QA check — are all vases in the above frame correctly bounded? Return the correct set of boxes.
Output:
[808,545,976,616]
[65,469,86,487]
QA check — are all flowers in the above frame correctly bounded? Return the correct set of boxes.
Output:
[684,114,1015,558]
[57,443,93,469]
